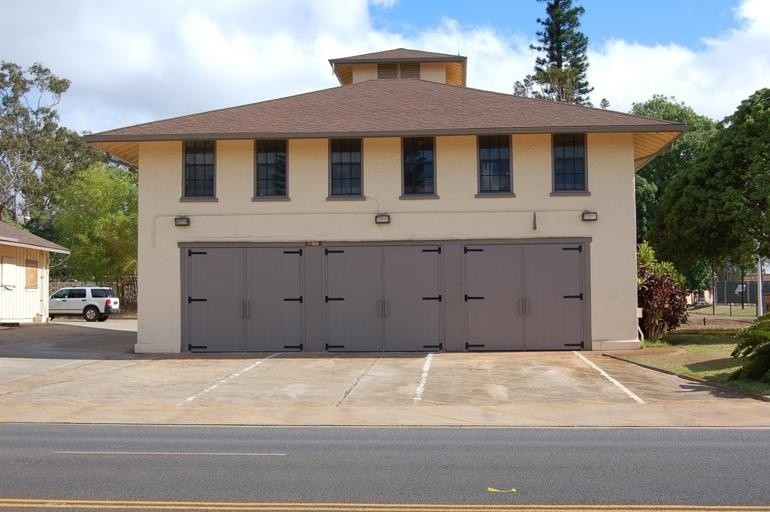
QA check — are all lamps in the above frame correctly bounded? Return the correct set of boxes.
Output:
[581,210,597,221]
[375,213,390,224]
[175,216,190,227]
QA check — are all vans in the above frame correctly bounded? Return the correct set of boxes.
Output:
[49,286,122,322]
